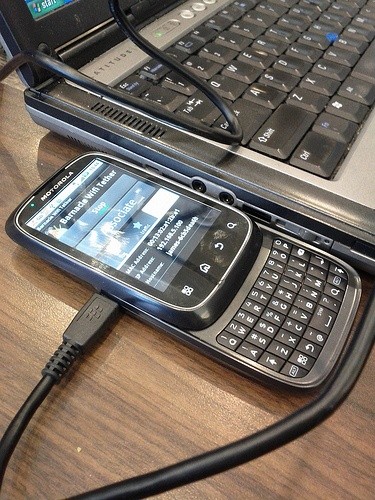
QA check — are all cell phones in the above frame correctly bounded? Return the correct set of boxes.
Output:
[5,150,362,389]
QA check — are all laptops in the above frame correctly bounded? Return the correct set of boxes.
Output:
[0,0,375,272]
